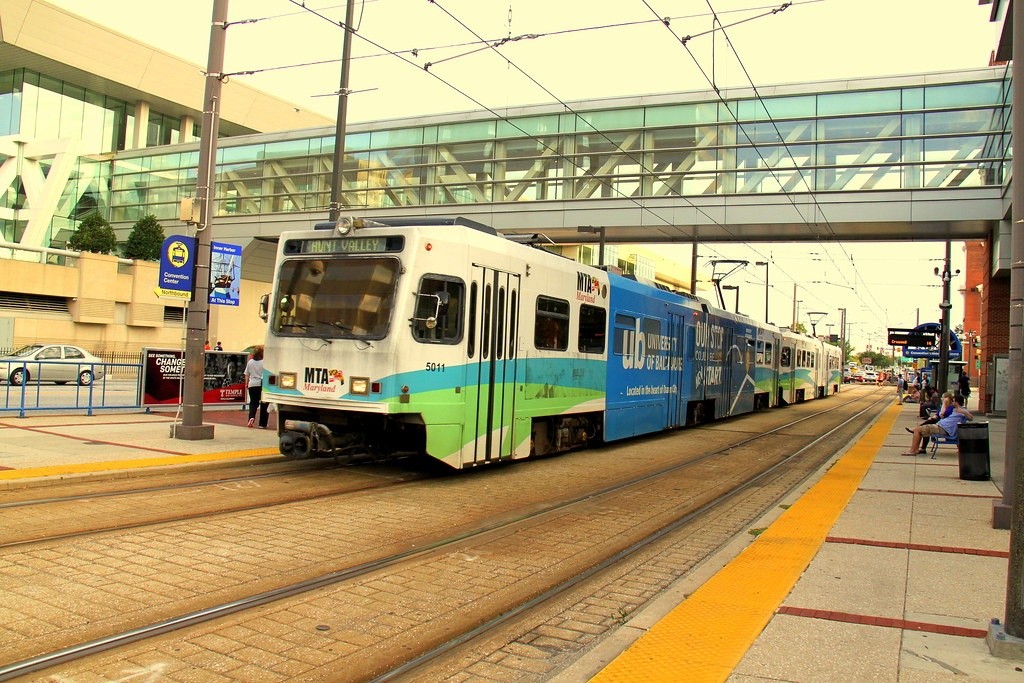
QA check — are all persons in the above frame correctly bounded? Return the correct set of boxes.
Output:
[877,371,883,387]
[956,371,971,408]
[222,355,244,388]
[214,342,223,351]
[901,392,974,456]
[243,345,269,429]
[204,340,209,349]
[897,372,941,420]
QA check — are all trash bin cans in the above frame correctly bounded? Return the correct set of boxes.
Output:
[954,420,993,481]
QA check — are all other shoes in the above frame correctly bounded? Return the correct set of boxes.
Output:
[922,415,930,420]
[905,427,914,433]
[247,418,254,428]
[259,425,265,429]
[897,403,902,405]
[918,450,926,454]
[901,451,916,456]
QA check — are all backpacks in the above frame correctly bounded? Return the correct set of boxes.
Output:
[961,380,971,395]
[903,380,908,390]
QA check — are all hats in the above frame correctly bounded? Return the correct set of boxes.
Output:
[897,374,902,376]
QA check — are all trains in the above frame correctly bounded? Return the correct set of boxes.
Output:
[258,214,842,472]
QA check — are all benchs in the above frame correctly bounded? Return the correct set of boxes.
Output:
[930,415,968,459]
[927,394,941,417]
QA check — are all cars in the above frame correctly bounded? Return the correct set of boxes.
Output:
[0,344,107,387]
[841,361,912,386]
